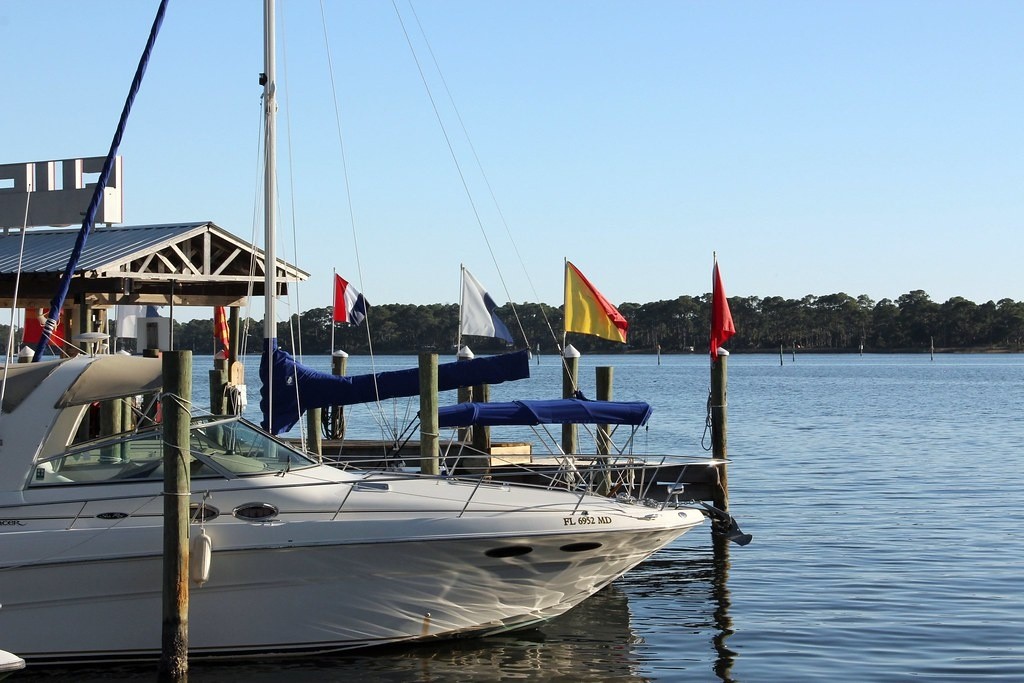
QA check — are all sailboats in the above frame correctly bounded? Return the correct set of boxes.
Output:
[0,0,755,678]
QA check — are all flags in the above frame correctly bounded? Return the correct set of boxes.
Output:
[711,266,735,360]
[116,303,164,338]
[334,273,369,327]
[566,263,626,344]
[214,306,230,357]
[461,271,513,342]
